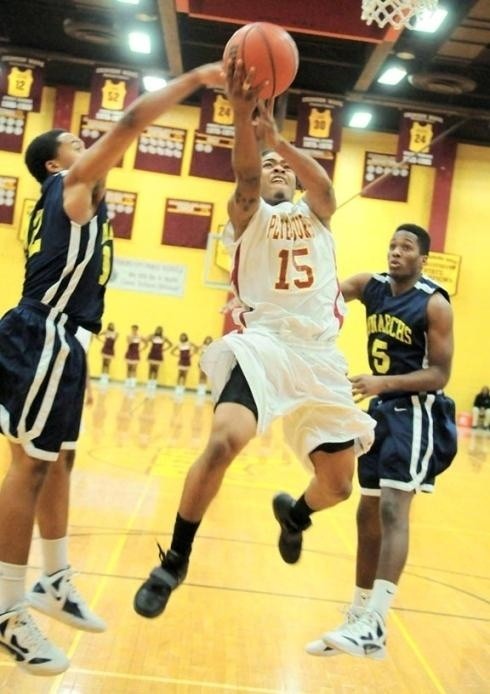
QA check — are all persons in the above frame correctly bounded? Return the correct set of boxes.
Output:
[198,336,218,396]
[125,324,148,389]
[302,226,458,654]
[135,49,378,620]
[145,327,172,390]
[171,333,198,395]
[97,321,119,386]
[0,60,237,677]
[470,385,490,431]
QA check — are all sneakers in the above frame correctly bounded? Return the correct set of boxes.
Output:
[0,607,70,676]
[134,548,190,618]
[306,607,387,661]
[272,491,302,564]
[28,565,106,633]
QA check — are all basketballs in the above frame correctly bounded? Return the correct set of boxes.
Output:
[224,22,300,100]
